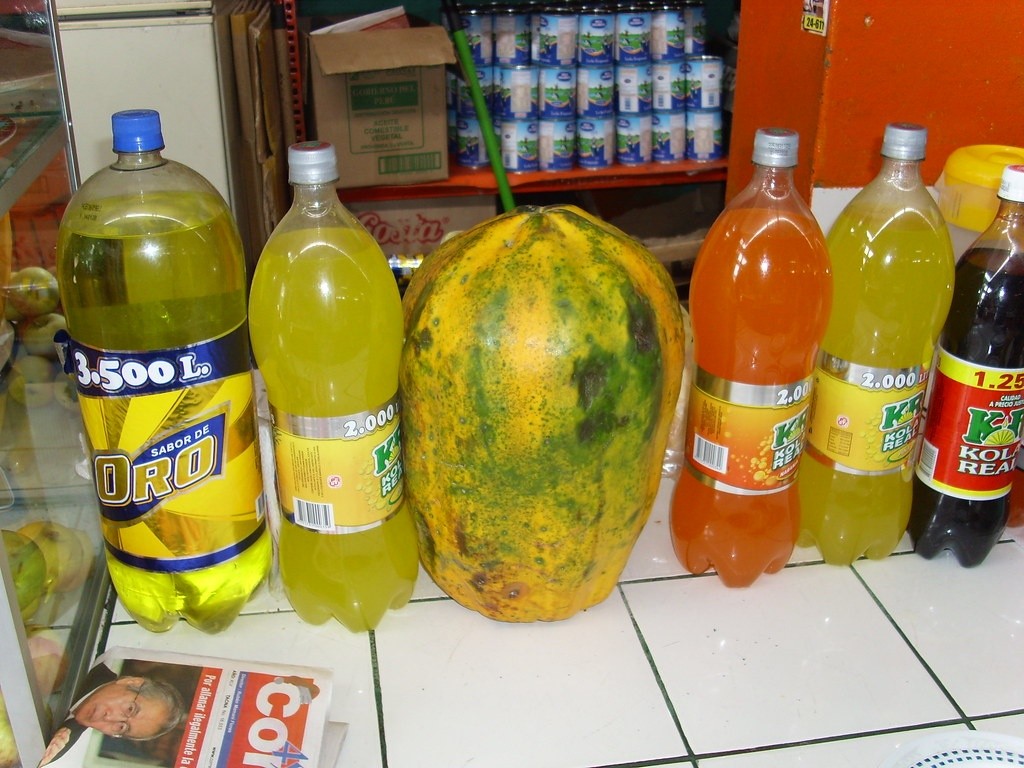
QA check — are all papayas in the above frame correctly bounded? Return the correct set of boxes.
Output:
[395,205,686,624]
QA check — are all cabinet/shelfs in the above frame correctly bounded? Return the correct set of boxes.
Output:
[279,0,838,293]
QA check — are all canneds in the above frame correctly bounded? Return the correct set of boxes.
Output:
[439,0,724,173]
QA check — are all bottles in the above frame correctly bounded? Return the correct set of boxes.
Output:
[795,122,954,564]
[247,141,418,631]
[671,128,832,587]
[56,109,273,631]
[907,165,1024,567]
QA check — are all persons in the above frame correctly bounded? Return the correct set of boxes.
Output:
[35,663,184,768]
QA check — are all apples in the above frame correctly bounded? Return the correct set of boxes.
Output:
[3,266,82,414]
[0,521,94,768]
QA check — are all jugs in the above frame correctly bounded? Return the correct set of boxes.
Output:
[934,144,1024,267]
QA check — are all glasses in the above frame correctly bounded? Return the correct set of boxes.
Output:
[112,681,146,739]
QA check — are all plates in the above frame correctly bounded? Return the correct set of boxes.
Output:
[0,116,17,146]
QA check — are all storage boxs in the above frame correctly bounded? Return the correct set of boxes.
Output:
[341,195,496,257]
[309,24,457,189]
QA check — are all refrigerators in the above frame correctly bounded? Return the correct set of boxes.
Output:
[55,0,256,291]
